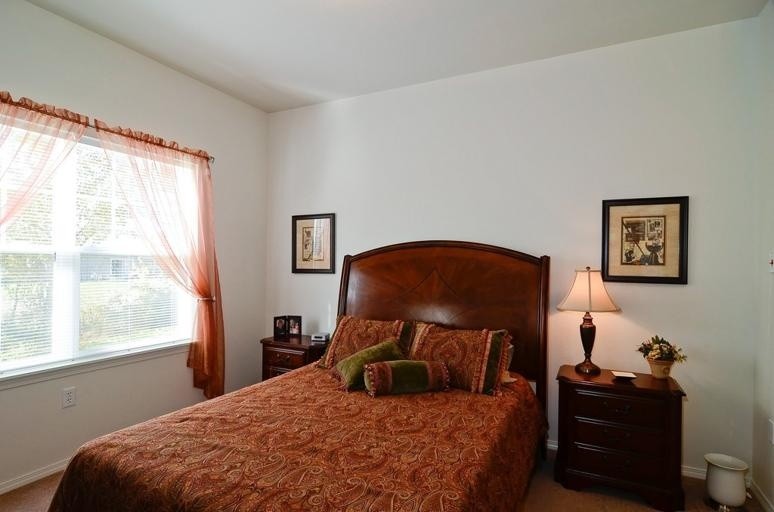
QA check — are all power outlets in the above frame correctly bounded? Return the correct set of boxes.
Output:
[61,384,76,408]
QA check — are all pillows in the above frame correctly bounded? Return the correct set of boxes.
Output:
[406,322,515,396]
[362,359,451,397]
[315,312,414,373]
[327,337,406,393]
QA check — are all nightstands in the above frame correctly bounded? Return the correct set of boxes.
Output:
[554,362,686,510]
[259,333,329,381]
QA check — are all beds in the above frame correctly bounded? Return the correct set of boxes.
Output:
[47,238,554,511]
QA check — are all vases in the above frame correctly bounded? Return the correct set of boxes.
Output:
[646,358,675,380]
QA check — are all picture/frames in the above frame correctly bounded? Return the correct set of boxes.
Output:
[600,195,690,285]
[287,314,302,344]
[290,211,337,275]
[272,315,288,340]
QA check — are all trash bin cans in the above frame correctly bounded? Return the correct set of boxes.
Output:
[704,453,753,512]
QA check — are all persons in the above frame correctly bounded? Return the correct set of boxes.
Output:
[276,320,284,333]
[290,322,300,335]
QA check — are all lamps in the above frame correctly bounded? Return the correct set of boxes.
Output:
[556,266,622,376]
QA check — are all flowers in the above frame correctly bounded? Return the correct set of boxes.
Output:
[636,334,686,362]
